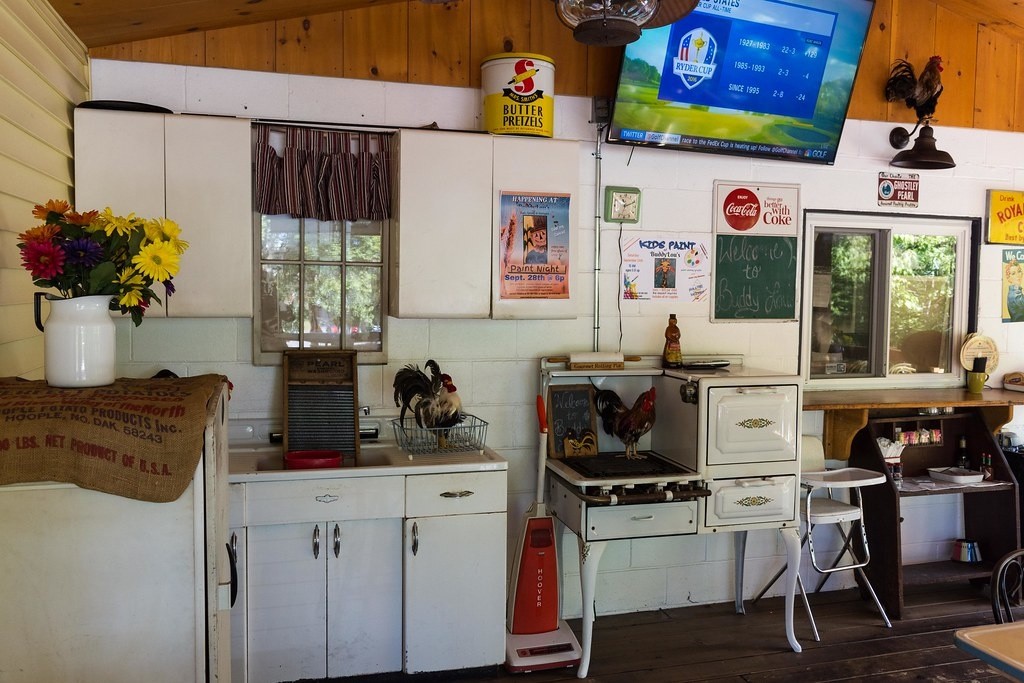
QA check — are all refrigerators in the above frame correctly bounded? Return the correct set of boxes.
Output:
[1,382,238,683]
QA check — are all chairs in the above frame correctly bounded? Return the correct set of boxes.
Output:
[991,549,1024,625]
[752,435,892,641]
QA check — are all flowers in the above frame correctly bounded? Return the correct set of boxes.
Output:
[18,198,191,326]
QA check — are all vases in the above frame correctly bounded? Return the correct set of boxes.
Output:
[34,292,116,388]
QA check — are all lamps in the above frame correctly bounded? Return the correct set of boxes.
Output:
[552,0,700,46]
[604,186,641,224]
[890,118,956,170]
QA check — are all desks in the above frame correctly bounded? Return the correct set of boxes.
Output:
[954,622,1024,683]
[539,351,803,678]
[803,388,1024,621]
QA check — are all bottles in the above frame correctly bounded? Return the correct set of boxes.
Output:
[957,435,969,469]
[886,461,904,491]
[662,313,683,369]
[979,451,995,482]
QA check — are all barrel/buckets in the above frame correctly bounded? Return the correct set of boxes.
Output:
[479,53,556,138]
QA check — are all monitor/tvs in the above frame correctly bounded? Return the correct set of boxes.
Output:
[605,0,877,166]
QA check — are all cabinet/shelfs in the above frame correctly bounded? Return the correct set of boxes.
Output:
[231,469,507,683]
[388,128,578,320]
[73,107,253,318]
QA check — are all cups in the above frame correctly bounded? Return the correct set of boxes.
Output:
[968,372,989,392]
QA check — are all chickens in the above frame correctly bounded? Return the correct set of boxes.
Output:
[592,380,659,466]
[883,54,954,124]
[393,359,465,454]
